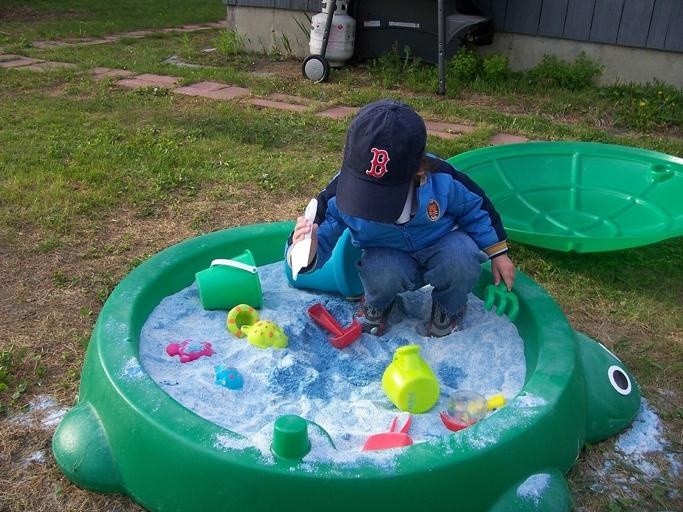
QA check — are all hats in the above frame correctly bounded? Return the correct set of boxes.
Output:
[336,98,427,223]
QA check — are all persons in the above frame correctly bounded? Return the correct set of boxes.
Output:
[282,99,515,338]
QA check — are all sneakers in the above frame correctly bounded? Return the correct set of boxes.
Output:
[352,296,384,335]
[430,301,466,338]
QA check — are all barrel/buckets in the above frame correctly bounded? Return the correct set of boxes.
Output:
[195,247,263,309]
